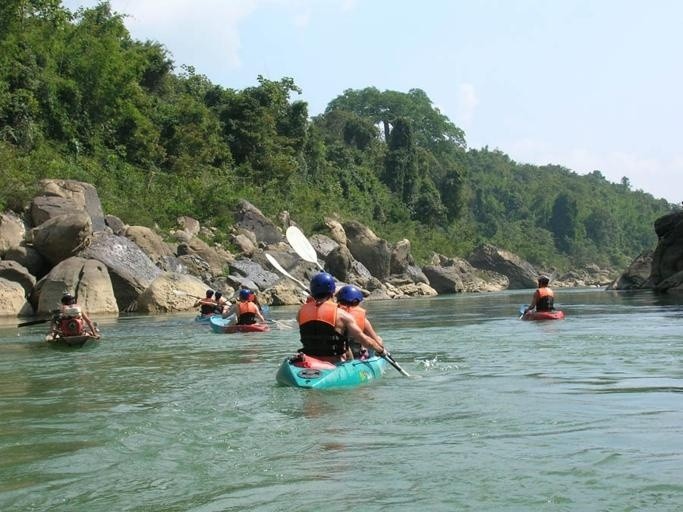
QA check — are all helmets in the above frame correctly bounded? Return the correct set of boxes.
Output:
[206,290,222,296]
[61,295,73,304]
[336,285,363,306]
[240,289,250,297]
[310,273,335,298]
[537,275,549,283]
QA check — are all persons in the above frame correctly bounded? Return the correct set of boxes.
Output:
[193,289,218,317]
[222,289,264,325]
[525,276,555,313]
[334,285,383,360]
[51,295,96,338]
[296,272,387,363]
[215,291,227,315]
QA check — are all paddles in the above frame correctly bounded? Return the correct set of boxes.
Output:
[265,226,408,376]
[519,271,560,319]
[225,289,242,302]
[173,290,200,299]
[18,320,51,327]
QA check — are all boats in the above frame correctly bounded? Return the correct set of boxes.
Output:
[208,315,273,334]
[273,350,389,392]
[194,303,270,325]
[520,303,566,321]
[43,328,102,350]
